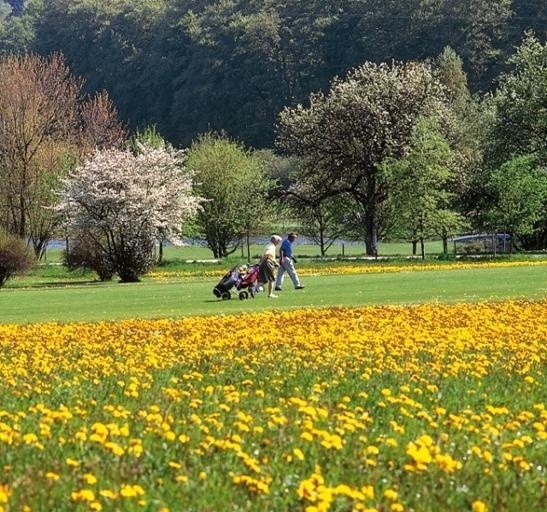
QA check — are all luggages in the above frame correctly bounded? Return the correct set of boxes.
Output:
[213,263,260,300]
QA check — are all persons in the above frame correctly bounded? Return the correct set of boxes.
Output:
[259,234,282,298]
[275,232,306,290]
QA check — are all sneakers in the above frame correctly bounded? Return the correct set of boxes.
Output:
[275,285,305,291]
[268,294,278,299]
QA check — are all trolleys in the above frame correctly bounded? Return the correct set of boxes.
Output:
[213,264,264,302]
